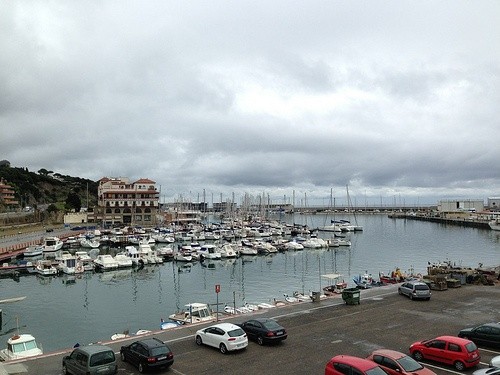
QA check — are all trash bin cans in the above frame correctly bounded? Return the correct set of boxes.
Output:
[312,292,320,301]
[342,288,360,305]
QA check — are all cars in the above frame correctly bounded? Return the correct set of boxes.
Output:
[70,226,87,231]
[408,335,481,373]
[65,237,76,242]
[234,318,288,345]
[325,355,388,375]
[398,281,432,301]
[457,323,499,352]
[118,337,174,375]
[365,350,437,375]
[46,228,54,232]
[194,323,248,355]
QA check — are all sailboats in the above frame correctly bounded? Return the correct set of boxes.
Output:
[362,196,414,213]
[159,184,363,263]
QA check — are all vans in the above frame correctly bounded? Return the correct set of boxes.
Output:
[125,225,144,233]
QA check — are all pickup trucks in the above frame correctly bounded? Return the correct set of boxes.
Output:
[110,228,123,235]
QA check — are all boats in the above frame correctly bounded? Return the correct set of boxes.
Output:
[169,303,215,324]
[0,334,44,363]
[111,330,131,341]
[135,328,153,336]
[43,236,64,251]
[353,270,380,288]
[488,218,500,231]
[215,291,328,315]
[23,245,44,257]
[323,274,348,297]
[79,239,103,248]
[0,229,174,276]
[380,266,415,285]
[160,320,181,330]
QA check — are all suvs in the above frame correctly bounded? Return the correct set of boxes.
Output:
[62,343,119,375]
